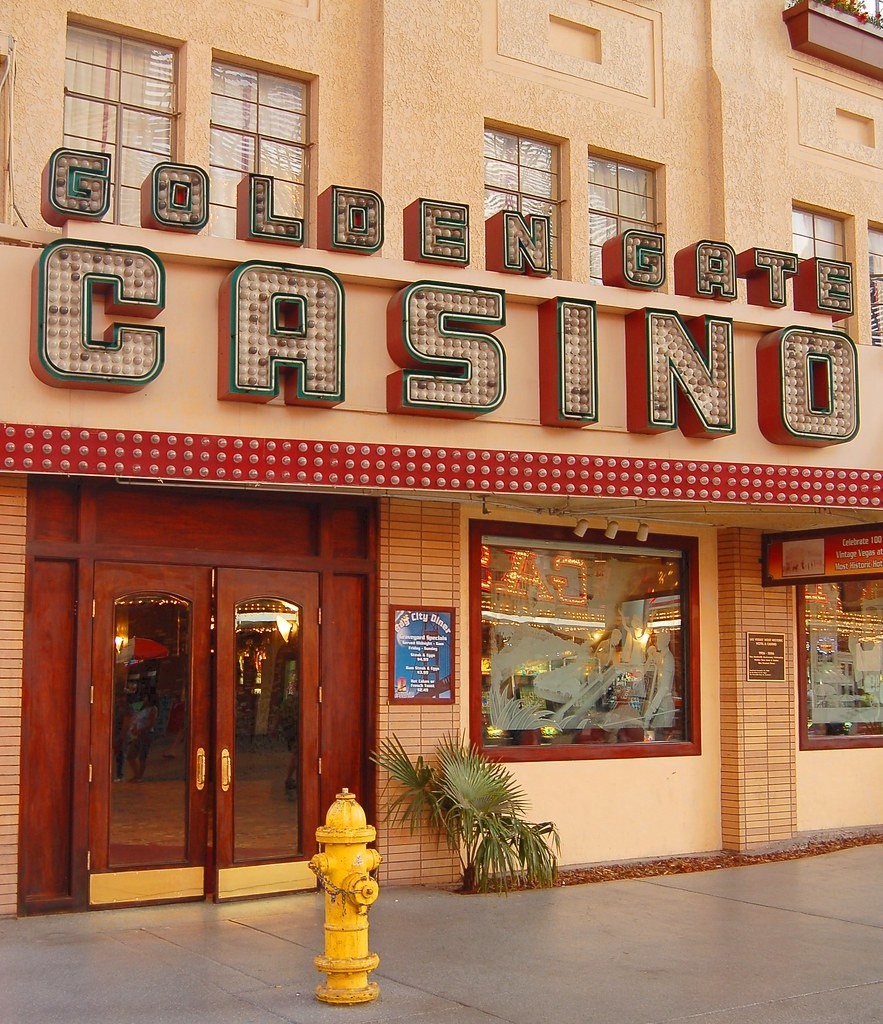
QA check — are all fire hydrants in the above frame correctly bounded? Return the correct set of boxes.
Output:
[304,789,384,1006]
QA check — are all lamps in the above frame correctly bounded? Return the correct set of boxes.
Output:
[636,523,649,541]
[573,519,589,538]
[605,521,619,539]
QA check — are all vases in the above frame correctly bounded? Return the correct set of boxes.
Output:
[783,0,883,81]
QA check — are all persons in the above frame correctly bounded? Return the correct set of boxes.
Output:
[271,679,300,799]
[113,688,185,783]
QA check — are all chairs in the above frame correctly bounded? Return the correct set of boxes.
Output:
[518,728,644,745]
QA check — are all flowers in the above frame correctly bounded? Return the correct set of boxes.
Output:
[788,0,883,29]
